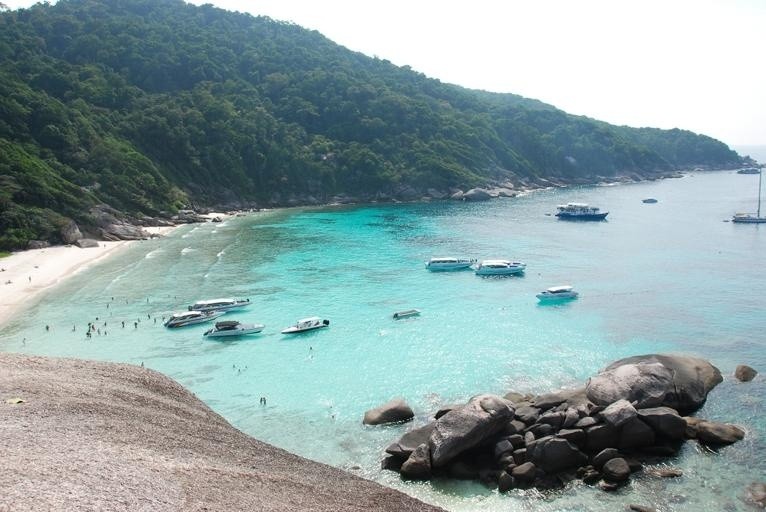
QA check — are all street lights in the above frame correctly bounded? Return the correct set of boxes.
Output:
[642,199,657,203]
[535,286,577,298]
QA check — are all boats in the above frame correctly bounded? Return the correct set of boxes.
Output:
[424,257,526,275]
[281,316,328,333]
[732,211,766,224]
[555,203,608,219]
[394,309,421,318]
[164,298,265,336]
[737,168,760,174]
